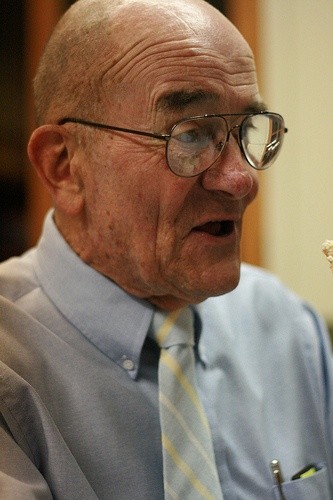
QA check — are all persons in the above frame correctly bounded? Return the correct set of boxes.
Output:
[1,0,333,500]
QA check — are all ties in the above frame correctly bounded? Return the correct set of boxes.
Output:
[148,309,223,499]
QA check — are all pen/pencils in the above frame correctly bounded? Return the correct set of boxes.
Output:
[271,460,287,500]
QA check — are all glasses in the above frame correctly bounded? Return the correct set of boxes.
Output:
[58,111,289,178]
